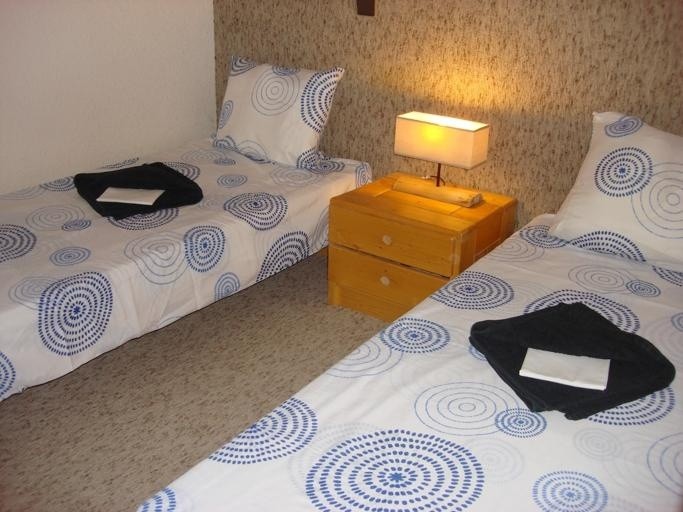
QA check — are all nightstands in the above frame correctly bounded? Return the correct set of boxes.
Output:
[327,172,518,325]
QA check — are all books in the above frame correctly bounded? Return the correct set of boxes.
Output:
[519,346,614,393]
[95,186,168,207]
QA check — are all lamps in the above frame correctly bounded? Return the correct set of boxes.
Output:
[394,111,490,208]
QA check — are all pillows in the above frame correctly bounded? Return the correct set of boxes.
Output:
[213,56,346,173]
[547,110,683,275]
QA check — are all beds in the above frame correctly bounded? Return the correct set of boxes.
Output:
[0,137,370,400]
[136,212,683,512]
